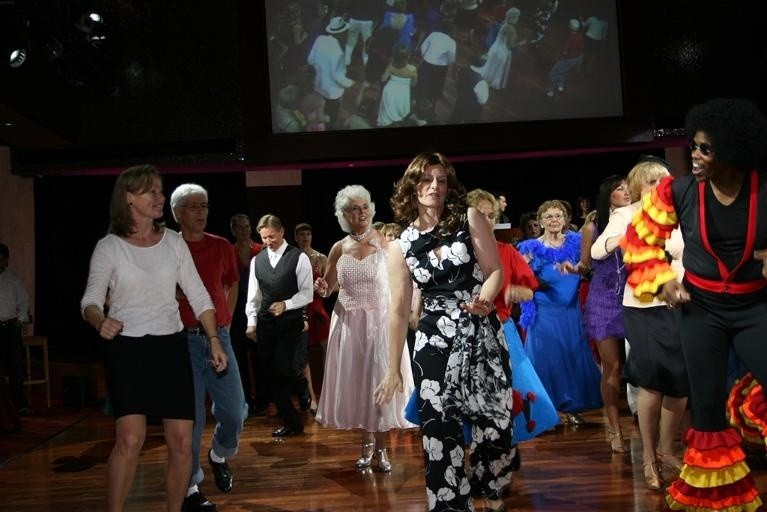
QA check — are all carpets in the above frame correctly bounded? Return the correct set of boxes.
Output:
[0,360,107,473]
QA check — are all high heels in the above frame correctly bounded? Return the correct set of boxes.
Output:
[355,437,377,469]
[643,454,665,492]
[565,413,585,426]
[607,428,630,454]
[655,451,683,477]
[375,448,393,472]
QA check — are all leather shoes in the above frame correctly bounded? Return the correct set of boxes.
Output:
[207,447,235,493]
[271,418,304,437]
[295,378,312,412]
[180,491,218,512]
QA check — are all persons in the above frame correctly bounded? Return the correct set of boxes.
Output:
[81,164,230,512]
[171,183,247,512]
[373,152,513,511]
[623,102,766,512]
[315,186,418,471]
[404,189,561,503]
[232,174,628,454]
[270,1,609,139]
[0,241,36,417]
[591,161,686,489]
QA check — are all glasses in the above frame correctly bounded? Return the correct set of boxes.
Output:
[176,202,208,212]
[689,139,719,157]
[540,213,565,220]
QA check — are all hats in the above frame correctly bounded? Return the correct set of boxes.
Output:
[325,16,350,33]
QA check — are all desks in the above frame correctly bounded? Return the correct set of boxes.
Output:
[21,335,51,409]
